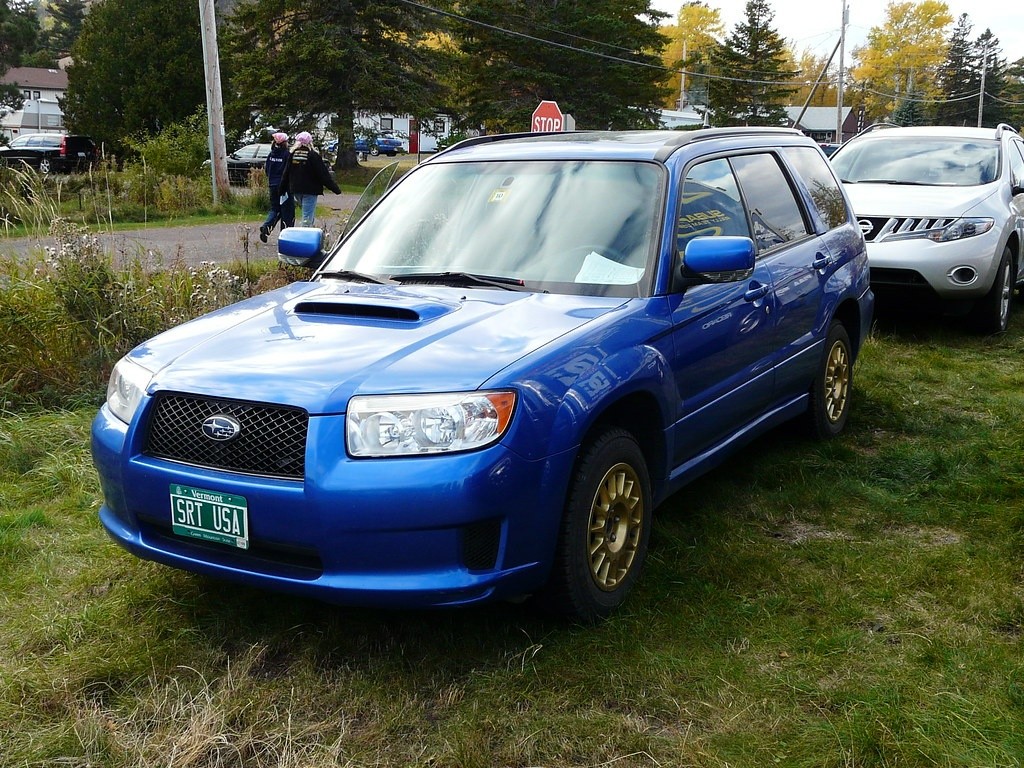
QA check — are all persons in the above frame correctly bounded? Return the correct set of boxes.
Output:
[279,132,341,227]
[260,133,295,243]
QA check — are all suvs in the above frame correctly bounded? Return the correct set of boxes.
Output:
[823,121,1024,337]
[88,126,881,613]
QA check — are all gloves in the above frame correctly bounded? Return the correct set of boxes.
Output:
[278,188,285,196]
[334,187,341,195]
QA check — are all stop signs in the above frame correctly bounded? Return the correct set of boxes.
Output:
[531,100,563,134]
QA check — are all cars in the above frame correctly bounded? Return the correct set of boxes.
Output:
[815,141,840,158]
[243,129,279,146]
[201,143,336,189]
[326,130,403,157]
[0,133,100,175]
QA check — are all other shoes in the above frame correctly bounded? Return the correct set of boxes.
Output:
[260,225,267,243]
